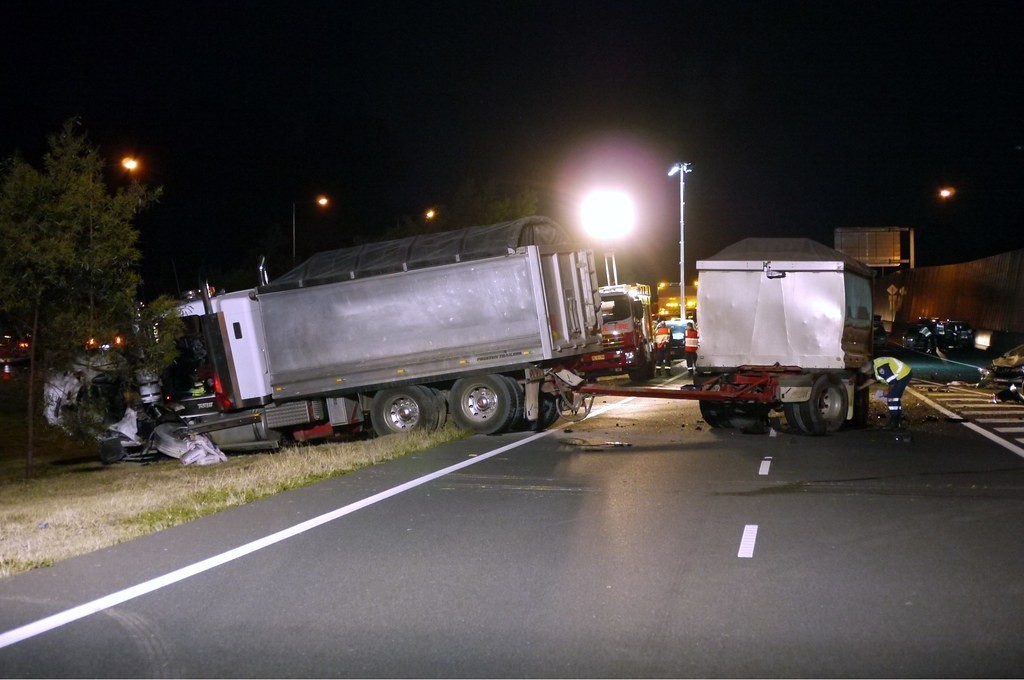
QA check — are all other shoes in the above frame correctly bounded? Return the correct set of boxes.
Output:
[688,370,693,376]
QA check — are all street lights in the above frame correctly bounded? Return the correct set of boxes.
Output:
[668,163,693,317]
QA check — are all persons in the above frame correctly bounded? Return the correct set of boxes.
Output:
[858,356,913,429]
[684,322,698,376]
[655,320,672,371]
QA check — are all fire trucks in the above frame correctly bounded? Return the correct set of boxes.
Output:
[578,284,657,382]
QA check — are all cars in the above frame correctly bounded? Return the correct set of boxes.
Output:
[945,320,974,345]
[873,324,888,348]
[902,323,937,351]
[655,319,696,357]
[980,341,1024,403]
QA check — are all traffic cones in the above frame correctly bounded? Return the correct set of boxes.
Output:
[2,359,12,380]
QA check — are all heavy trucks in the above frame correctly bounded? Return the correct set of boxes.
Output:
[144,215,607,453]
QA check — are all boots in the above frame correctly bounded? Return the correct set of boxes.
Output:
[665,370,672,377]
[656,369,661,376]
[881,416,902,431]
